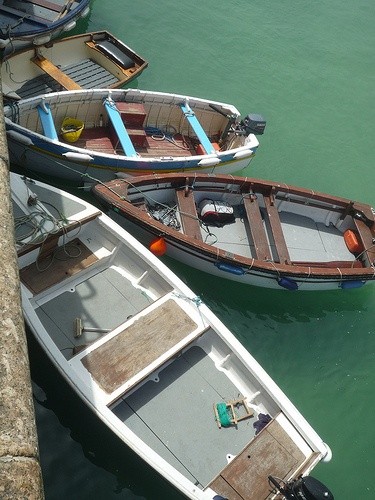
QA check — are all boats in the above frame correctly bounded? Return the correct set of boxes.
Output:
[0,0,91,50]
[0,87,268,188]
[82,164,375,295]
[0,27,147,106]
[5,168,340,500]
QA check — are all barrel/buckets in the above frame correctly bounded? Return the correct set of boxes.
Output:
[62,117,84,142]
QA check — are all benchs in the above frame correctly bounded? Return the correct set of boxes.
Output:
[201,408,313,500]
[352,216,375,269]
[68,288,211,406]
[84,37,141,81]
[18,229,116,305]
